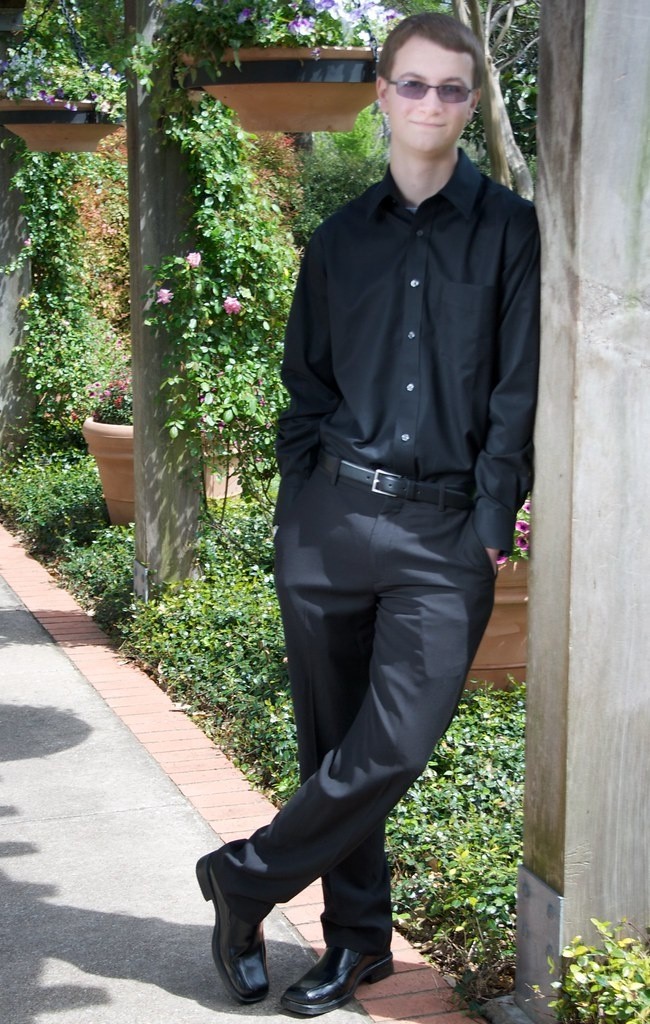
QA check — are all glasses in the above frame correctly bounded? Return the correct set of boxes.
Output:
[388,80,474,103]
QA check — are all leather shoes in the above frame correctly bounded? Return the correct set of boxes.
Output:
[281,946,394,1015]
[196,850,269,1003]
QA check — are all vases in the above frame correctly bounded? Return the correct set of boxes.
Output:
[0,99,120,152]
[462,559,529,698]
[82,417,253,527]
[178,46,378,134]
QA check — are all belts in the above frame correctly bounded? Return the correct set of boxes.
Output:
[316,449,475,509]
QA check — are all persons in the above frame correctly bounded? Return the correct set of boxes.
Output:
[196,13,541,1015]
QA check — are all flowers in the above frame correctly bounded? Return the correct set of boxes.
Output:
[70,364,135,426]
[0,42,126,124]
[126,0,408,146]
[497,493,530,568]
